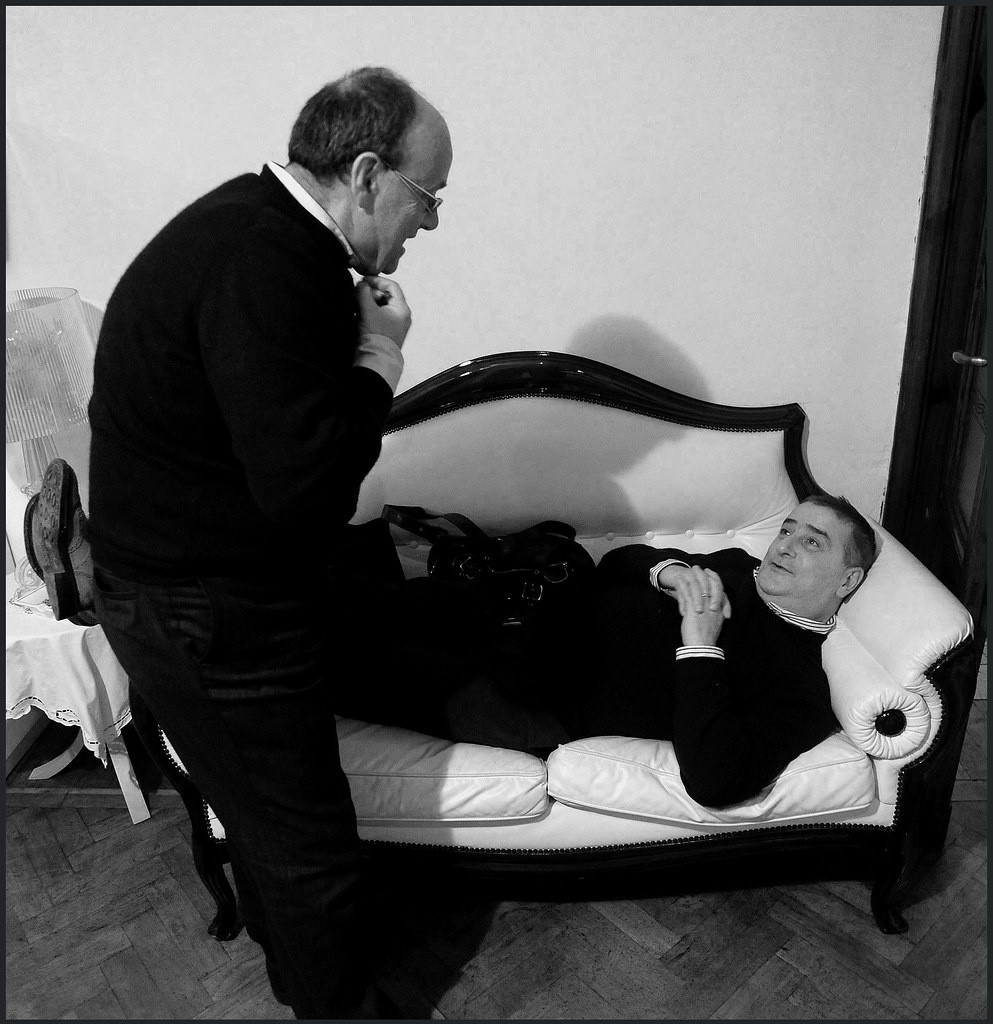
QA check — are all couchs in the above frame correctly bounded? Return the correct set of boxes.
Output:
[128,352,986,954]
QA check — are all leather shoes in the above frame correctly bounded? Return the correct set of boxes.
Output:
[24,457,101,626]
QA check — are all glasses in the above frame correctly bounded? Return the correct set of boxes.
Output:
[388,164,444,218]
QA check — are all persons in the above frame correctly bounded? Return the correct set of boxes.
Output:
[25,456,878,813]
[92,63,455,1024]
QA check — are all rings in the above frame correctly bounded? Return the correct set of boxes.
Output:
[701,593,710,600]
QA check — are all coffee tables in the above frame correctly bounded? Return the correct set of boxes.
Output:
[5,570,157,826]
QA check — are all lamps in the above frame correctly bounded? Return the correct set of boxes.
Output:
[5,286,108,619]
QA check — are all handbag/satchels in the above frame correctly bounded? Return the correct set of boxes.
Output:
[380,502,599,603]
